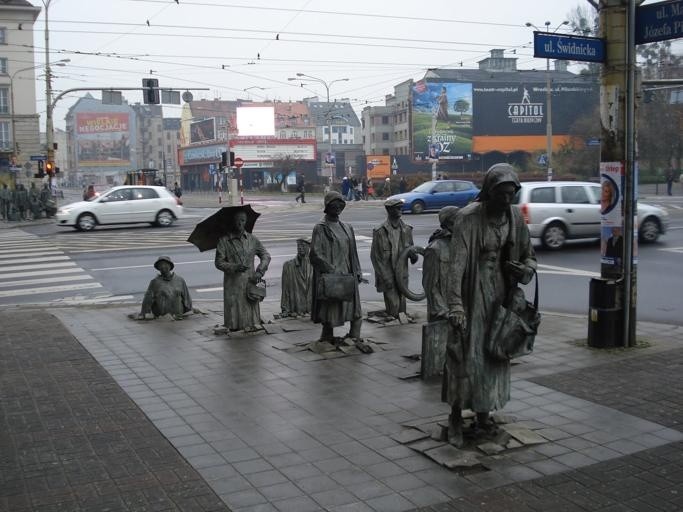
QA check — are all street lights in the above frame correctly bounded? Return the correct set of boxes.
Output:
[0,58,71,185]
[524,20,569,181]
[287,72,350,185]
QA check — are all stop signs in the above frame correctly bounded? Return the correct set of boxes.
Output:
[233,158,243,168]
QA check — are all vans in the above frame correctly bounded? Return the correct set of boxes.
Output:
[510,180,669,250]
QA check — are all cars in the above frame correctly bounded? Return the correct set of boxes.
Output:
[55,185,184,232]
[385,179,481,213]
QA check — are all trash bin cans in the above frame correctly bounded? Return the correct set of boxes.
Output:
[322,185,330,196]
[588,277,622,347]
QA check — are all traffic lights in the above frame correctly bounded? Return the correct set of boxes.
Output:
[55,168,59,173]
[44,163,52,175]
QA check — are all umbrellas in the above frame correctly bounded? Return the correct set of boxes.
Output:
[187,204,261,252]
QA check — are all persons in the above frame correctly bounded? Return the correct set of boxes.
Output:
[280,237,316,318]
[295,174,307,202]
[421,203,461,324]
[9,155,19,167]
[309,192,363,339]
[606,226,624,256]
[85,184,95,198]
[599,180,614,205]
[215,212,271,332]
[665,163,675,196]
[443,170,449,180]
[438,173,442,179]
[437,86,449,120]
[174,182,181,196]
[370,199,417,318]
[141,258,192,319]
[440,163,539,446]
[0,183,56,221]
[342,174,372,202]
[384,178,391,195]
[400,175,407,192]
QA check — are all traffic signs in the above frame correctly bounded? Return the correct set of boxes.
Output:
[533,31,605,63]
[635,0,683,44]
[30,156,46,160]
